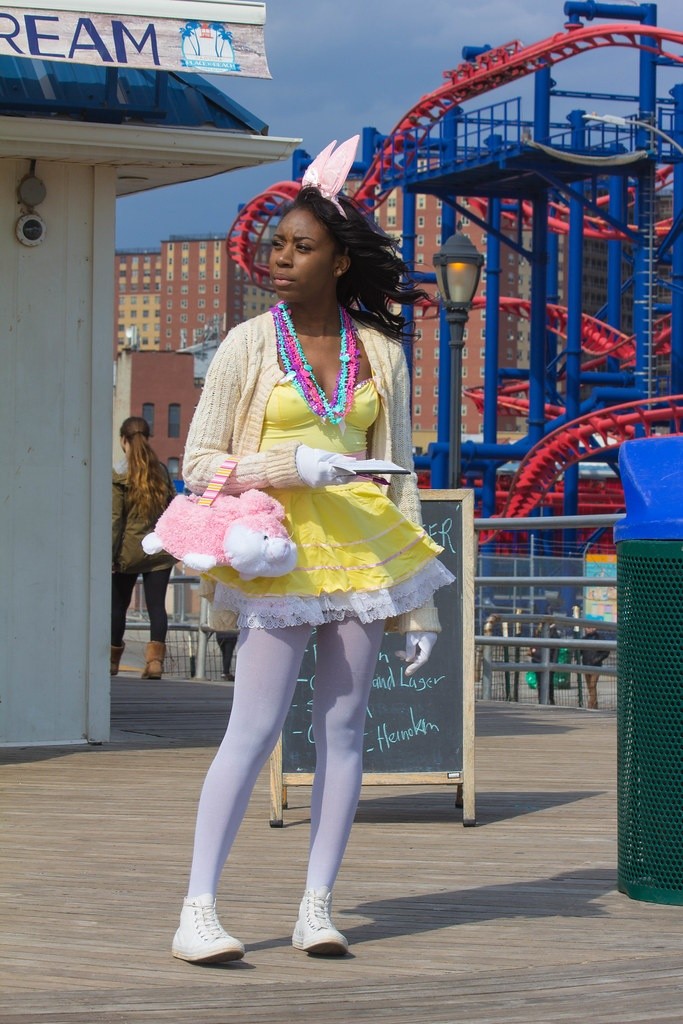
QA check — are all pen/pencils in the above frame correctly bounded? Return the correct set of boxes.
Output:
[356,472,391,487]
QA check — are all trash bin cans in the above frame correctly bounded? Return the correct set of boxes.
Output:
[526,650,571,690]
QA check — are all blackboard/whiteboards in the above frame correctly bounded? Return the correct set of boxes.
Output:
[271,490,476,787]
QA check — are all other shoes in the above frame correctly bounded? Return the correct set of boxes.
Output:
[220,672,234,681]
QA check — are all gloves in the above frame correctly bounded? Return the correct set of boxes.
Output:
[395,629,439,676]
[295,446,356,488]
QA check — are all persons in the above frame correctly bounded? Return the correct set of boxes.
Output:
[112,416,180,682]
[198,576,242,683]
[578,626,612,709]
[171,134,456,965]
[528,614,561,706]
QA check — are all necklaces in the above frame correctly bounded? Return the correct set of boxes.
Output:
[270,301,363,425]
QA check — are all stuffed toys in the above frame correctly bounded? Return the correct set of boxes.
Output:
[141,458,298,582]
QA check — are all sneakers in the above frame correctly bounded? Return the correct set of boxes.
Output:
[171,894,245,964]
[292,885,348,956]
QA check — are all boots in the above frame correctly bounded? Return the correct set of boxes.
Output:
[141,641,168,681]
[110,640,127,676]
